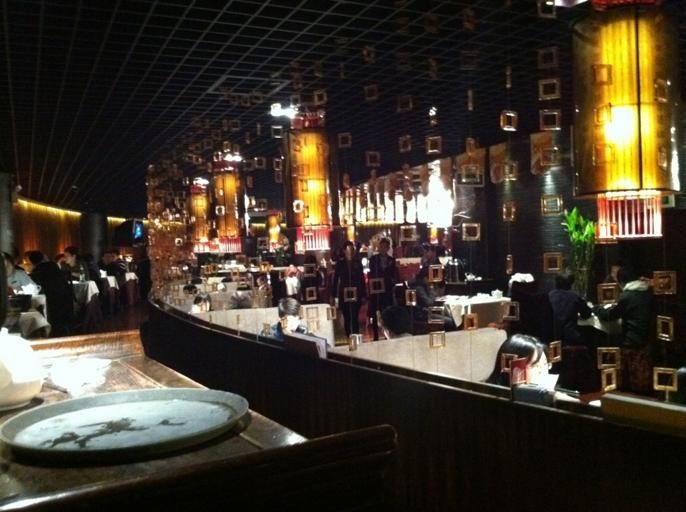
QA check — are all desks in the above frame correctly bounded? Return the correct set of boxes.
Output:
[436,294,512,328]
[9,272,139,338]
[0,327,408,512]
[577,302,625,340]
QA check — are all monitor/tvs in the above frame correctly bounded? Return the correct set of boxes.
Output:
[114,219,145,242]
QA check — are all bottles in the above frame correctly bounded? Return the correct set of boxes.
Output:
[80,265,85,282]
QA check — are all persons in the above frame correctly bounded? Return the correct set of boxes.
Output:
[548,271,605,350]
[603,263,623,284]
[0,236,151,338]
[175,234,469,344]
[483,332,555,387]
[589,264,656,355]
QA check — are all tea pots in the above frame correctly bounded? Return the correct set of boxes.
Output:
[22,283,42,295]
[491,288,503,299]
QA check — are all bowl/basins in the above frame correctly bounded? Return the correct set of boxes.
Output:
[0,365,48,413]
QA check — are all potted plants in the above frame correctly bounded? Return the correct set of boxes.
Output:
[561,208,595,298]
